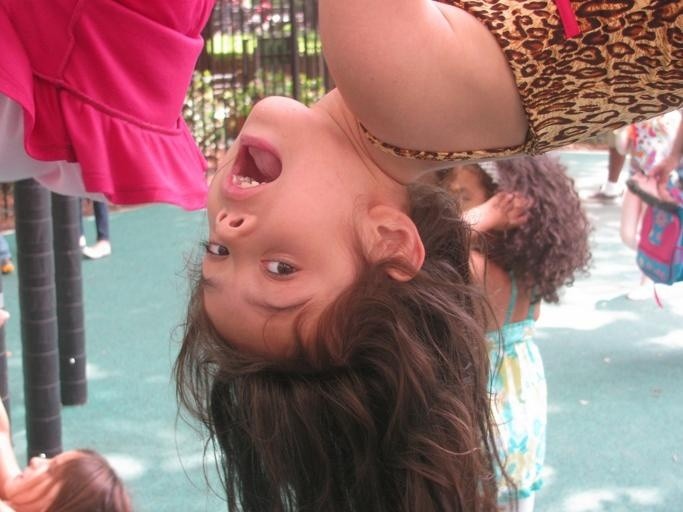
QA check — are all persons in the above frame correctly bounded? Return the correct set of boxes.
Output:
[167,0,683,511]
[0,0,219,213]
[587,109,683,286]
[76,196,112,259]
[453,148,596,511]
[0,395,130,512]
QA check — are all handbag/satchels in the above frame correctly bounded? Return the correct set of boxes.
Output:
[636,187,683,285]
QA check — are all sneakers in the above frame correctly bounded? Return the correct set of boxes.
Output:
[82,242,110,258]
[626,171,677,211]
[585,186,623,204]
